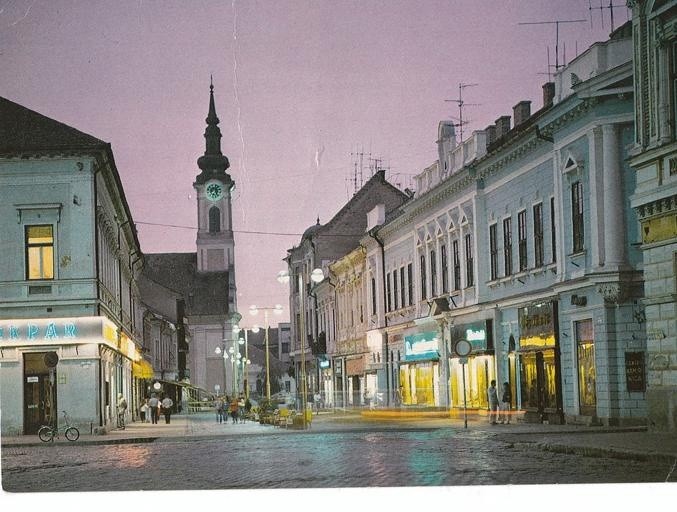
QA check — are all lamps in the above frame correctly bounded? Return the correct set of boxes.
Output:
[571,295,587,306]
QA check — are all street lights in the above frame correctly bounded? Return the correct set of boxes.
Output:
[214,303,285,414]
[274,269,325,430]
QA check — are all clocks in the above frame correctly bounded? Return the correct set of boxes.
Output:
[205,184,224,202]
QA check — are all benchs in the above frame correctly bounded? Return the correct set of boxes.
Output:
[274,416,288,428]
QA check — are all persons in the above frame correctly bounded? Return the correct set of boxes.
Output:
[118,393,127,430]
[363,388,369,406]
[395,386,403,412]
[213,396,251,424]
[488,380,499,424]
[500,382,512,424]
[177,399,182,413]
[314,390,325,415]
[139,393,174,424]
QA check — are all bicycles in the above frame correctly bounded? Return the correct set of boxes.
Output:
[37,411,80,442]
